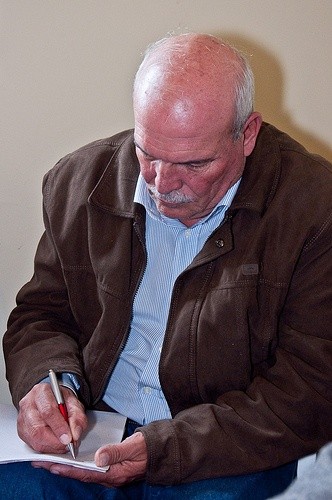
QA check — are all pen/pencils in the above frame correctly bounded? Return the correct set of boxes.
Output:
[48,368,76,460]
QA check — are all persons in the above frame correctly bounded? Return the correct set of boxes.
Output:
[2,30,330,500]
